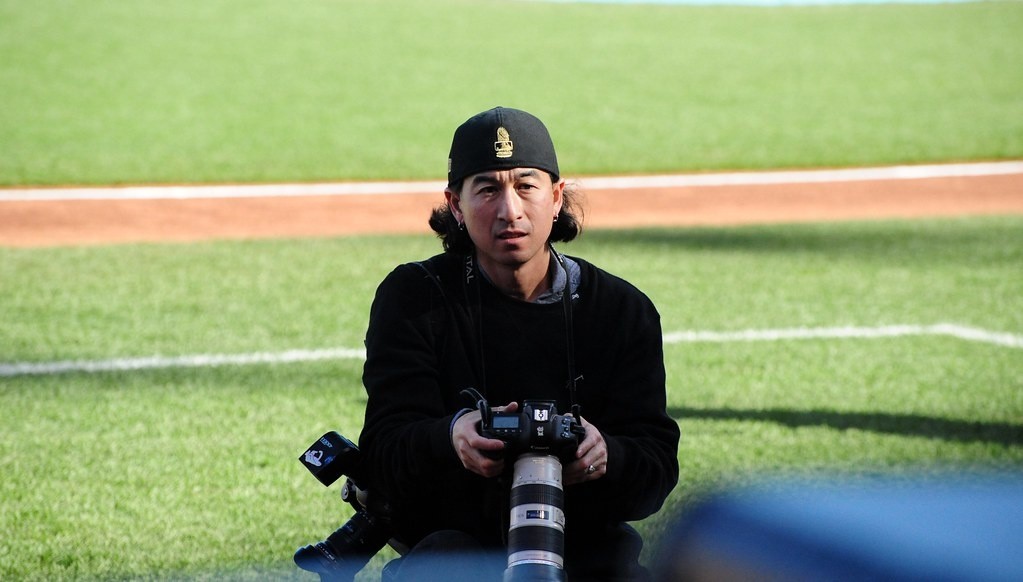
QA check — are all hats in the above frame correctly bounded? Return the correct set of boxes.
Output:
[448,106,560,186]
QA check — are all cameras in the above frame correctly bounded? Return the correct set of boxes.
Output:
[477,398,586,582]
[294,431,402,582]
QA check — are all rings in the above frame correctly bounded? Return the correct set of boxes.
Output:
[588,465,596,473]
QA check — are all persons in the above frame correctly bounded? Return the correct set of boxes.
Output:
[357,106,680,582]
